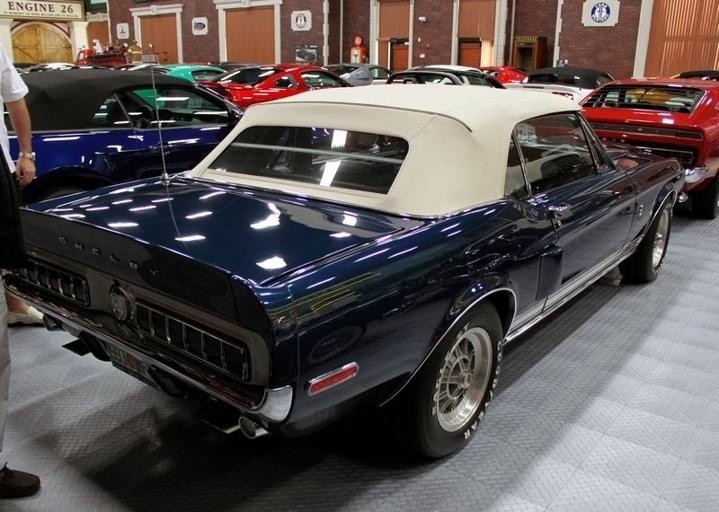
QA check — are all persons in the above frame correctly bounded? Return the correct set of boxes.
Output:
[0,278,40,498]
[0,47,49,325]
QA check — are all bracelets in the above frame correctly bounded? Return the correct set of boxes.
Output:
[19,150,36,161]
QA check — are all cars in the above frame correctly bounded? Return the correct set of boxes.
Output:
[2,60,719,221]
[2,83,690,464]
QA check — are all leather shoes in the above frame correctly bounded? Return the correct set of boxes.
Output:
[0,468,39,499]
[6,306,43,326]
